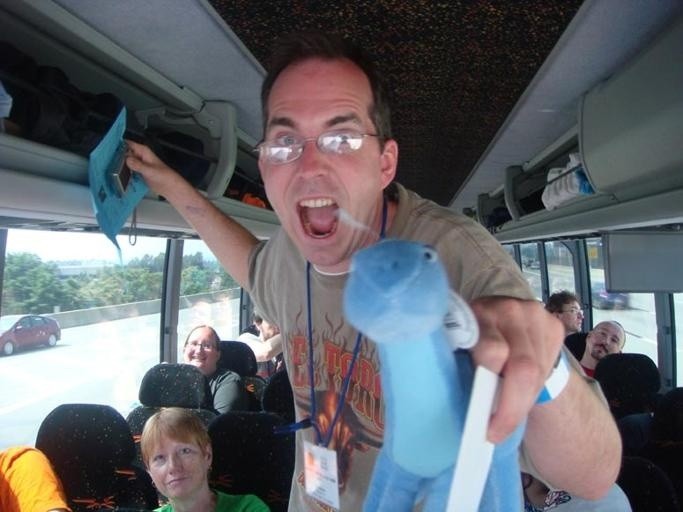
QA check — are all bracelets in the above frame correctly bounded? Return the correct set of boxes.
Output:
[536,349,571,406]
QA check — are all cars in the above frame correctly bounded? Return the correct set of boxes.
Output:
[520,255,541,271]
[588,280,631,312]
[0,313,61,356]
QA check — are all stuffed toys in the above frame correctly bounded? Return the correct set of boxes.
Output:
[341,239,525,511]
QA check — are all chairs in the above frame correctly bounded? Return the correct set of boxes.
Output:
[125,363,221,468]
[262,369,295,423]
[36,404,158,512]
[618,388,682,512]
[564,333,622,361]
[217,342,267,411]
[594,353,664,456]
[207,413,295,488]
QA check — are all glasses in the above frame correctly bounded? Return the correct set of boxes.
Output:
[252,128,380,165]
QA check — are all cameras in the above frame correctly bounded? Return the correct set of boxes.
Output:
[106,140,138,198]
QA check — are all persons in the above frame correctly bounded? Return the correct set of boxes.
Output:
[0,447,74,512]
[140,406,271,511]
[184,326,251,414]
[546,290,683,512]
[124,32,623,510]
[239,312,285,377]
